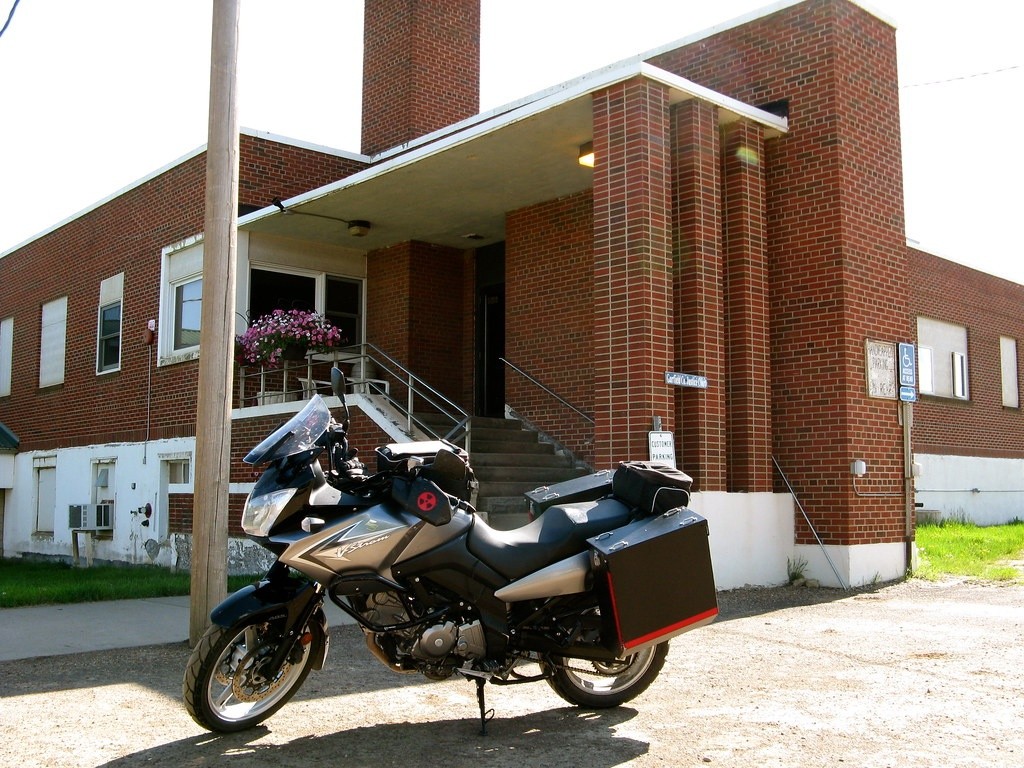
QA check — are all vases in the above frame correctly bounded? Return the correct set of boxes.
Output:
[257,392,297,406]
[280,341,308,361]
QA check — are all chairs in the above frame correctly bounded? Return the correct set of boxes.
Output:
[348,377,390,404]
[298,378,336,400]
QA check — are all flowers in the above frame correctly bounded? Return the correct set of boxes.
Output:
[234,309,348,369]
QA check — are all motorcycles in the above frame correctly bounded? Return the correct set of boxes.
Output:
[182,365,720,736]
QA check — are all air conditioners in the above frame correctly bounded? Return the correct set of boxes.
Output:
[68,504,114,530]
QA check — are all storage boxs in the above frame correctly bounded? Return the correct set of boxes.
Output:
[587,505,720,659]
[524,468,618,522]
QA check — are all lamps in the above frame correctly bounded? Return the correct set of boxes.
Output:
[348,220,371,236]
[579,140,594,167]
